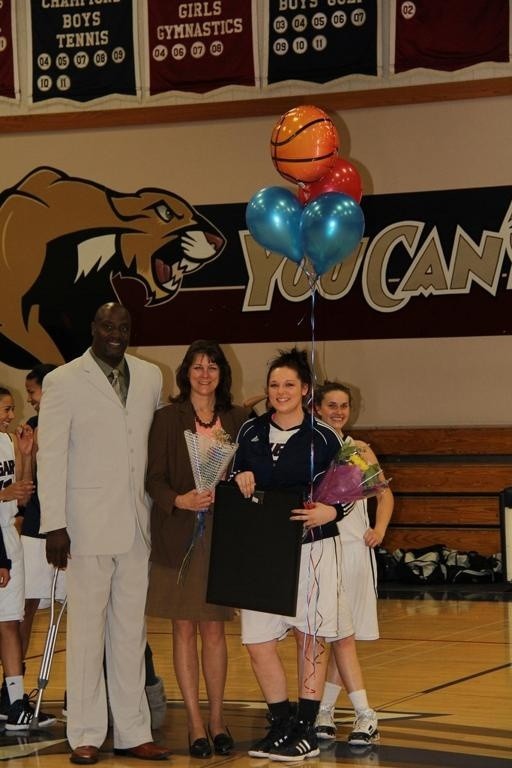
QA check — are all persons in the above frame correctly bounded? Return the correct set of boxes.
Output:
[148,338,259,758]
[60,638,165,729]
[1,363,66,730]
[37,301,178,764]
[239,352,354,762]
[308,378,399,743]
[243,390,274,419]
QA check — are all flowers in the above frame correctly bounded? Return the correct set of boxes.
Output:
[176,426,241,583]
[305,435,397,507]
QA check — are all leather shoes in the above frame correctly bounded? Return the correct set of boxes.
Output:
[188,728,211,758]
[114,741,172,758]
[207,722,234,754]
[70,745,99,763]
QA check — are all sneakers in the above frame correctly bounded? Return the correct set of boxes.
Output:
[348,710,380,744]
[5,693,57,730]
[248,700,337,760]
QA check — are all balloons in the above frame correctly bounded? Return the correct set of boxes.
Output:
[246,102,367,273]
